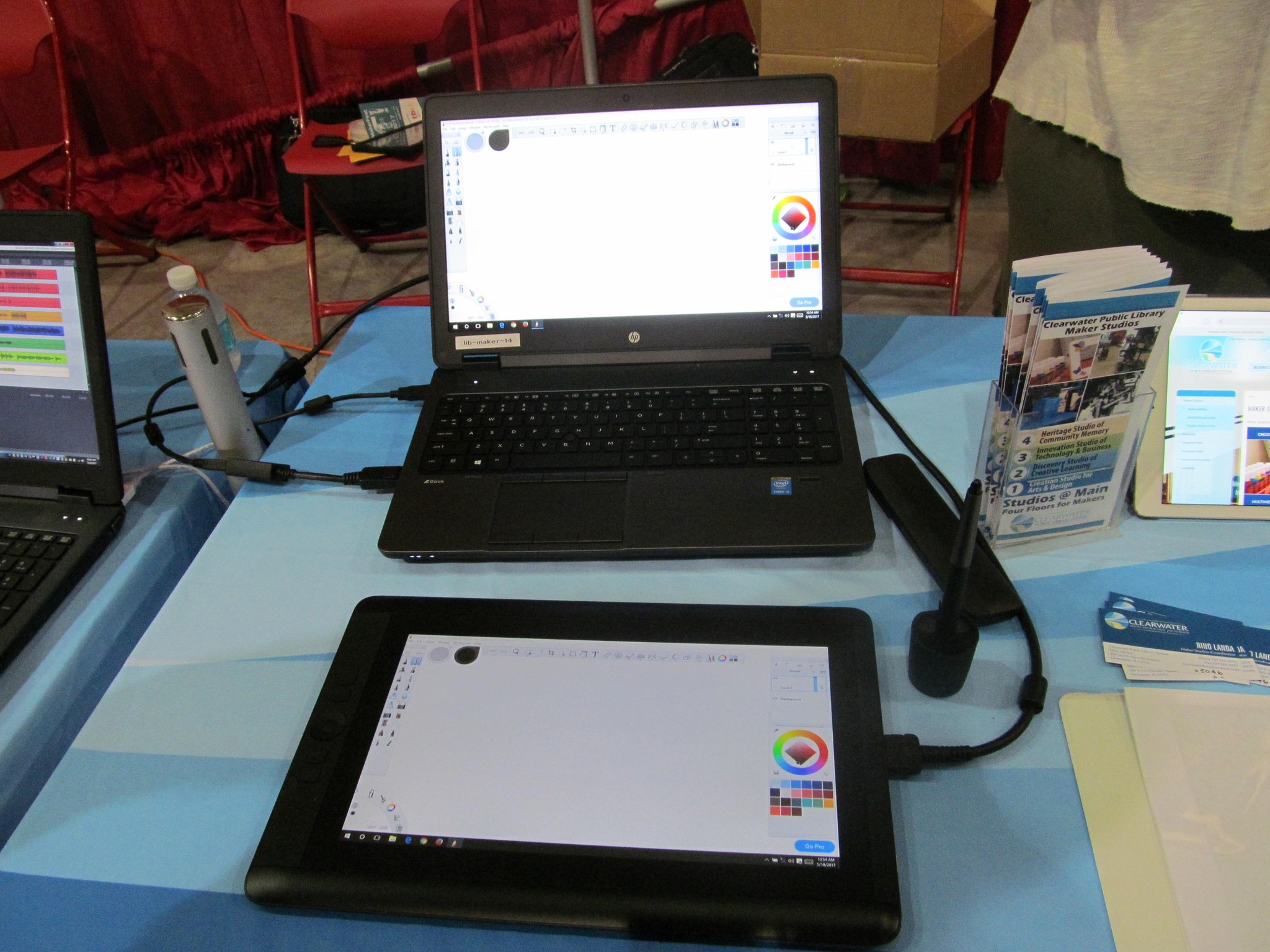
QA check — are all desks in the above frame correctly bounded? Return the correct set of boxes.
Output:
[0,335,308,847]
[0,307,1270,952]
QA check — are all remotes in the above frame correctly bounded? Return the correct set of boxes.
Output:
[863,454,1024,625]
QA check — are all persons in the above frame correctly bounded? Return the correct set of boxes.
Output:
[990,0,1270,317]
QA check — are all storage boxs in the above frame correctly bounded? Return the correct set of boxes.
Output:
[744,0,997,142]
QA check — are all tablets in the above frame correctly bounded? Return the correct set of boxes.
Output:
[1132,296,1270,521]
[245,593,901,952]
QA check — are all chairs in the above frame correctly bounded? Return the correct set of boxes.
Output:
[0,0,158,261]
[283,0,485,347]
[836,93,981,317]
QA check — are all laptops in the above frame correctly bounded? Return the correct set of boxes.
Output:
[1,208,127,669]
[376,74,869,564]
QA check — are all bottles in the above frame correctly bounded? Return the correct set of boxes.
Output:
[166,263,241,374]
